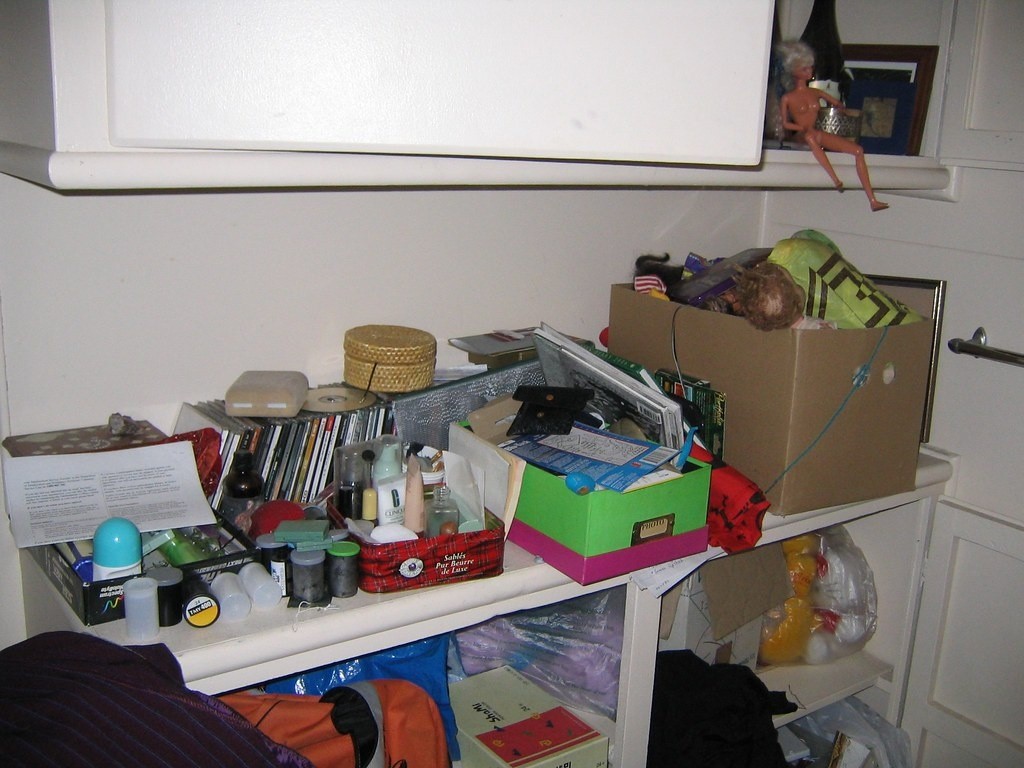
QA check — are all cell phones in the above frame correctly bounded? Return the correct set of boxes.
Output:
[673,247,773,303]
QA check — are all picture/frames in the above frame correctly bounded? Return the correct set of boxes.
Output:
[841,41,940,156]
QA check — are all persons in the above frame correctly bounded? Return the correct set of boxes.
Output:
[735,260,834,333]
[776,42,888,212]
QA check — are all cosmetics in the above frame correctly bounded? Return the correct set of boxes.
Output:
[329,439,485,544]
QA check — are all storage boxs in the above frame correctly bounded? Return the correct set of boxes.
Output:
[453,419,710,587]
[26,505,258,628]
[324,483,507,594]
[608,282,935,517]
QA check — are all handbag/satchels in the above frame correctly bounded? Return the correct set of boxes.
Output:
[646,649,798,768]
[215,633,461,768]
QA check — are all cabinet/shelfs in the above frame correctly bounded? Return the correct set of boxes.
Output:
[0,0,950,190]
[0,450,953,768]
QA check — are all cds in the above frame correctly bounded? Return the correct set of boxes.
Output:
[301,388,376,413]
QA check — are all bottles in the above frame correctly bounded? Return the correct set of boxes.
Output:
[223,452,266,534]
[424,486,459,539]
[122,534,360,640]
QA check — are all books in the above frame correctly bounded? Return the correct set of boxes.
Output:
[171,389,399,511]
[448,318,714,542]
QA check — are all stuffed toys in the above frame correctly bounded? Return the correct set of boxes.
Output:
[759,531,877,665]
